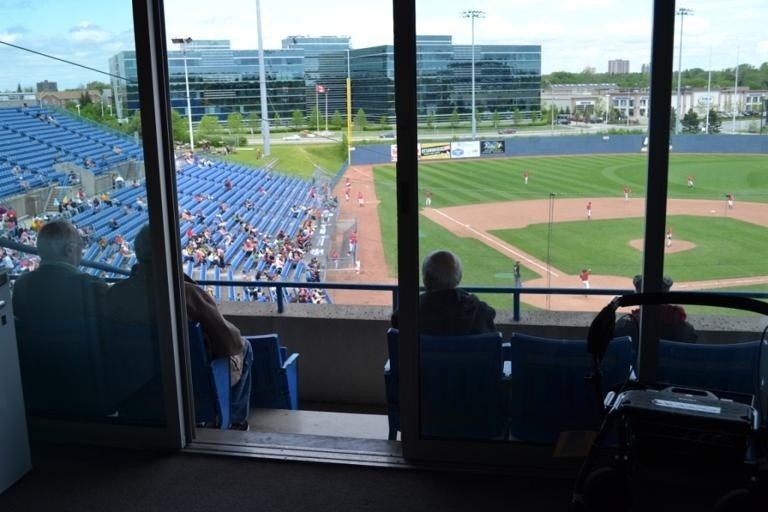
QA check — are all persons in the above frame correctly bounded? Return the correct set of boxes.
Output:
[102,223,255,434]
[686,175,696,191]
[727,190,735,210]
[666,223,673,247]
[586,201,593,221]
[1,102,366,303]
[10,220,109,332]
[522,167,529,186]
[511,259,522,288]
[586,271,697,381]
[622,184,631,202]
[579,268,591,297]
[386,251,495,336]
[423,186,433,208]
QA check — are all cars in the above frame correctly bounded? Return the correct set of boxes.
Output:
[712,109,760,119]
[569,113,628,123]
[378,129,397,138]
[697,123,720,134]
[283,134,301,141]
[498,127,516,135]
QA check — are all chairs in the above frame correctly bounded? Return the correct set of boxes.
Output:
[238,333,303,409]
[653,337,768,415]
[514,330,635,446]
[383,326,512,446]
[15,319,110,420]
[101,320,233,431]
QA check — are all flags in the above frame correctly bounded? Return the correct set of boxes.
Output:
[315,84,329,94]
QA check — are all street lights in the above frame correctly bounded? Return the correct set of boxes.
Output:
[604,94,610,133]
[171,37,195,154]
[107,104,112,117]
[75,104,81,116]
[323,88,330,138]
[675,8,695,135]
[549,85,555,130]
[462,10,487,140]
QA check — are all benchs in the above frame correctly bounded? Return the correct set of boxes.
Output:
[0,105,340,318]
